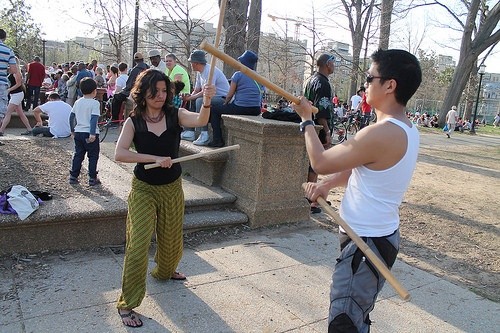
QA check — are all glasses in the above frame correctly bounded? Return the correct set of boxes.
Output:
[364,74,391,83]
[327,55,335,65]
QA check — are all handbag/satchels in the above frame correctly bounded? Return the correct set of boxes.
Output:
[443,123,448,131]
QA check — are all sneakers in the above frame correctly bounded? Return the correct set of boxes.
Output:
[20,130,32,136]
[180,130,195,141]
[193,131,210,145]
[209,140,225,147]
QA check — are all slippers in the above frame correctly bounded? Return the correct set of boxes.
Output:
[117,308,143,328]
[171,272,186,281]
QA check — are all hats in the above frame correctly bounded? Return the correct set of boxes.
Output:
[450,106,457,110]
[187,50,208,64]
[237,49,258,71]
[147,50,161,57]
[133,52,143,59]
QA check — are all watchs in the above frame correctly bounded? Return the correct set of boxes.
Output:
[299,119,315,134]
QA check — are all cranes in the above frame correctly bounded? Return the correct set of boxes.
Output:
[268,14,338,40]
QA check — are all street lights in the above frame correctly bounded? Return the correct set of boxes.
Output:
[469,62,486,134]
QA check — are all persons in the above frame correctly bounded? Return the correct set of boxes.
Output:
[163,53,190,109]
[68,76,102,186]
[114,68,216,328]
[206,50,264,147]
[301,53,336,213]
[148,48,167,72]
[0,57,131,121]
[0,29,17,127]
[179,49,236,146]
[27,92,73,139]
[0,56,33,136]
[102,51,150,128]
[331,86,500,141]
[259,88,296,114]
[291,48,423,333]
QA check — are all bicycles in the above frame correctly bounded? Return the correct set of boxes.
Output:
[345,108,361,140]
[349,111,375,130]
[329,109,346,146]
[95,96,114,143]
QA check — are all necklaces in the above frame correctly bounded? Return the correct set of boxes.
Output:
[141,109,165,124]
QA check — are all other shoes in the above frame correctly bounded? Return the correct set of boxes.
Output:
[68,175,79,183]
[89,179,101,185]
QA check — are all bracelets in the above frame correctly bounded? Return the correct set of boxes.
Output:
[325,129,331,135]
[202,103,211,108]
